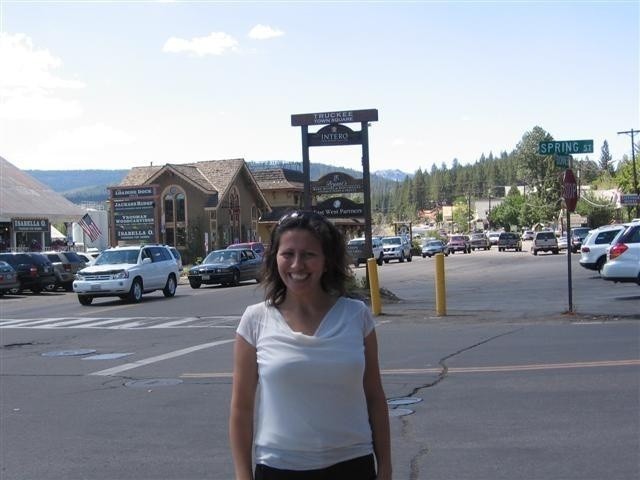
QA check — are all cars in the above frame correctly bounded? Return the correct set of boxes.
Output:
[601,218,640,285]
[188,248,268,287]
[226,242,266,259]
[420,239,450,258]
[487,233,500,245]
[556,233,569,251]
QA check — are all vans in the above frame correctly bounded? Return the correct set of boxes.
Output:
[345,235,385,266]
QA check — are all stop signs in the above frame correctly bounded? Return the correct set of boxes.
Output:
[562,168,581,214]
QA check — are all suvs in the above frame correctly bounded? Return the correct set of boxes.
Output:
[565,226,593,253]
[72,245,181,304]
[447,235,472,254]
[0,244,186,295]
[469,233,492,251]
[497,231,522,251]
[578,218,640,275]
[528,231,559,254]
[380,234,414,263]
[520,230,535,240]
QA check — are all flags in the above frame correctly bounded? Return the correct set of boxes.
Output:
[78,213,102,243]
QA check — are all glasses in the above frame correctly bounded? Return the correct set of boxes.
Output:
[278,210,325,229]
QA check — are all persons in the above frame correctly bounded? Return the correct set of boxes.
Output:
[229,209,393,480]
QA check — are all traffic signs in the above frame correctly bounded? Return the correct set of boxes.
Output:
[538,139,594,156]
